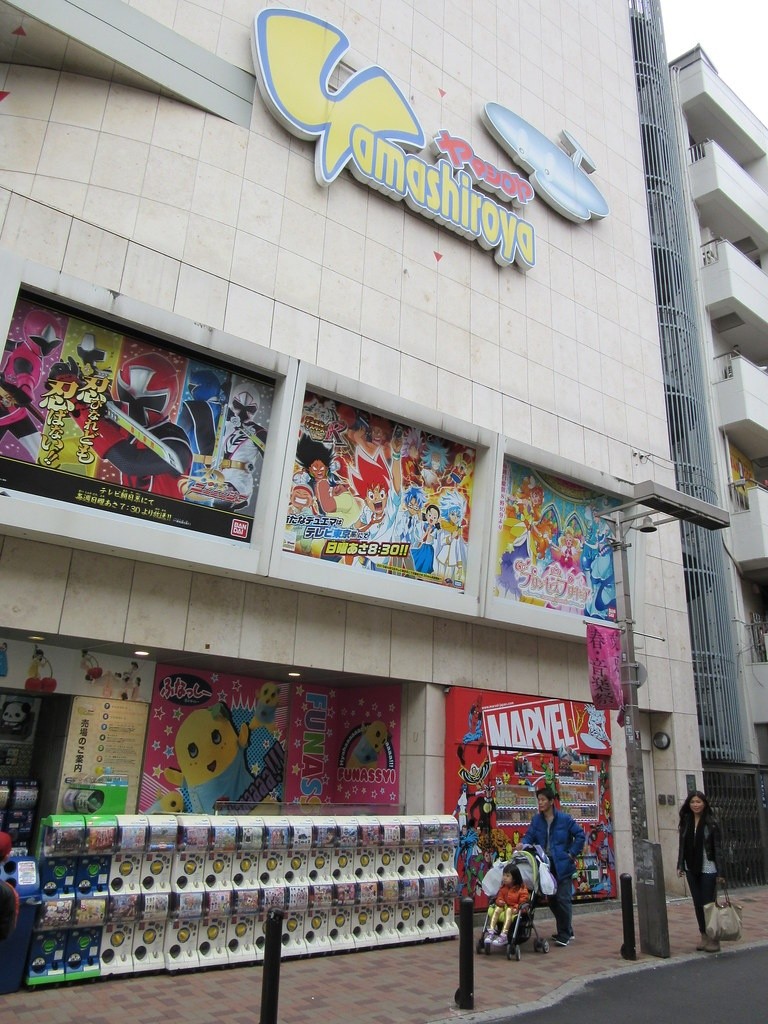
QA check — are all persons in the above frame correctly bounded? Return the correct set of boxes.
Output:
[0,832,19,942]
[515,788,586,946]
[677,790,728,952]
[483,865,532,946]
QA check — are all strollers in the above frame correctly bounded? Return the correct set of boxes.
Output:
[474,849,550,962]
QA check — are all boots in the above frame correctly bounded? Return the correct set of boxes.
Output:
[696,933,708,950]
[705,941,719,951]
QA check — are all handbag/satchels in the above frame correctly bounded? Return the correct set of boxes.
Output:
[535,854,557,896]
[703,882,743,941]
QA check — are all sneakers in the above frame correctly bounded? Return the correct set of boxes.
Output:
[491,935,508,946]
[551,932,575,939]
[484,933,495,943]
[556,936,569,946]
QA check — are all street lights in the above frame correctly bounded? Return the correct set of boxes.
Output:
[593,479,732,960]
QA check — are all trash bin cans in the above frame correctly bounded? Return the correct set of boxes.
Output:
[1,857,42,994]
